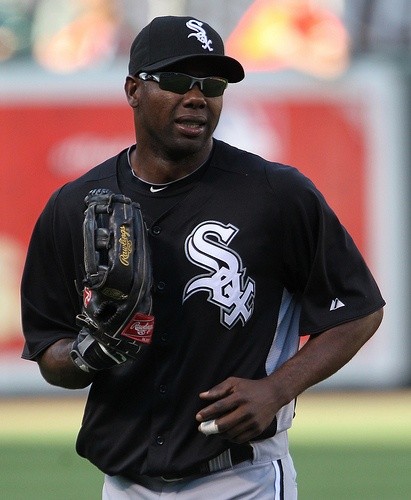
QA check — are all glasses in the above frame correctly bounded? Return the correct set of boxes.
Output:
[137,69,228,96]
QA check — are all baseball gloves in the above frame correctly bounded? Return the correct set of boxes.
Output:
[67,186,156,379]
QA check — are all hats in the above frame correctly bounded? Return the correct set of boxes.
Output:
[127,15,245,84]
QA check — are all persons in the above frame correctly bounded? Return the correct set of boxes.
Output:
[20,15,387,500]
